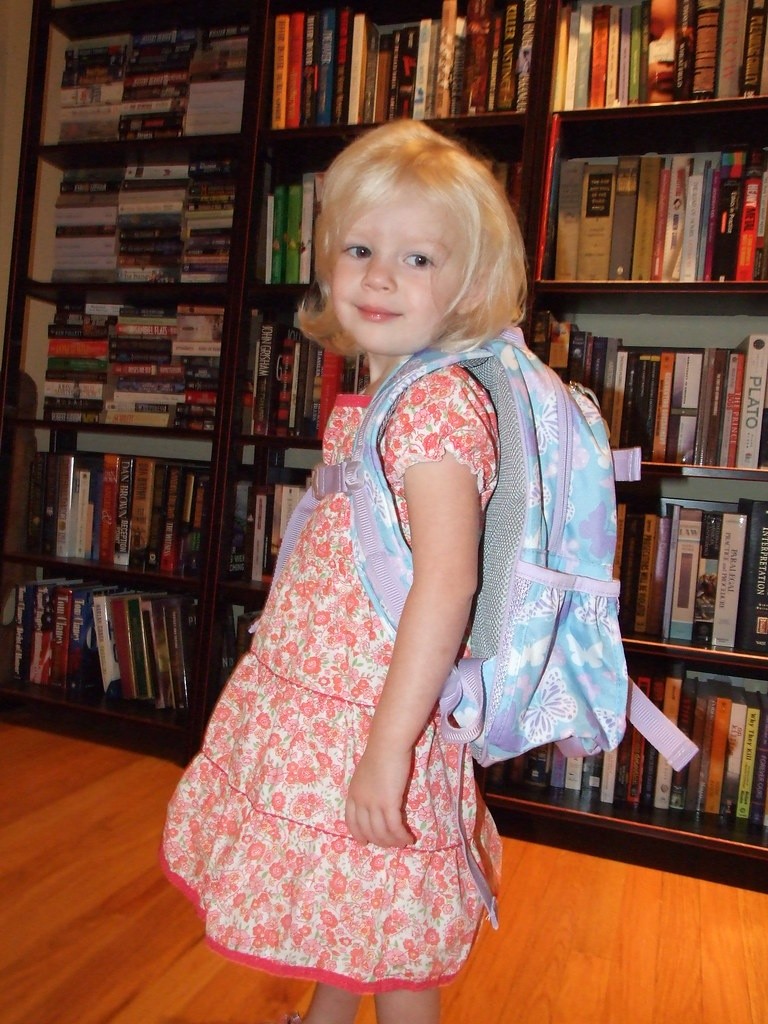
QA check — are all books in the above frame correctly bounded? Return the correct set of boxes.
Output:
[11,0,768,821]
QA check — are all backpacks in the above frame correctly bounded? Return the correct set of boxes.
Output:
[248,327,700,773]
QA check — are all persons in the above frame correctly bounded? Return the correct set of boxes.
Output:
[160,118,528,1024]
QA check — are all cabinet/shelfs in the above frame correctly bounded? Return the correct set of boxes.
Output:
[0,0,767,894]
